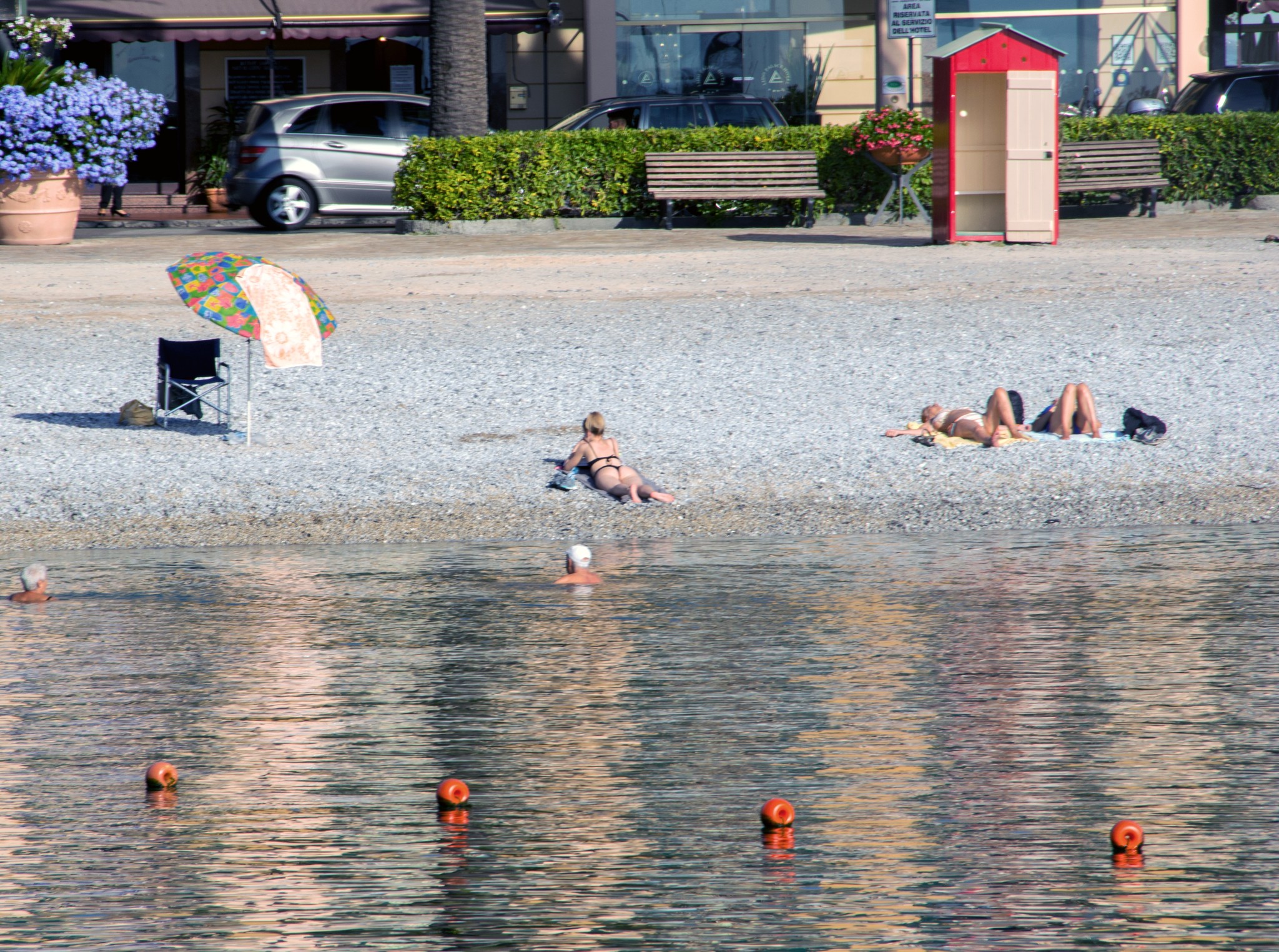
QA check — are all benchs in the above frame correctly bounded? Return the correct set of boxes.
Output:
[641,150,826,229]
[1057,137,1169,219]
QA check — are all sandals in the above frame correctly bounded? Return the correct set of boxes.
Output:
[1133,428,1162,445]
[913,429,934,446]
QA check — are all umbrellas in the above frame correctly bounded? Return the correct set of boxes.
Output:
[165,250,337,450]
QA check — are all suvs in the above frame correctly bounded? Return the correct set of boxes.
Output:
[224,91,514,233]
[1166,61,1279,115]
[547,91,792,136]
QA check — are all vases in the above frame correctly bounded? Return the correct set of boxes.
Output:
[0,162,87,247]
[866,143,930,164]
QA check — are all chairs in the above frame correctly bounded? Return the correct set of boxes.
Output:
[153,334,235,430]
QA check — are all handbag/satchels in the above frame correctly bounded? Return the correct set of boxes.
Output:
[119,400,155,426]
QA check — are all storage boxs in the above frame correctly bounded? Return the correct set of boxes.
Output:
[1126,97,1168,114]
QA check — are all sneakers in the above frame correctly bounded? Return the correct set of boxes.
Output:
[550,475,578,490]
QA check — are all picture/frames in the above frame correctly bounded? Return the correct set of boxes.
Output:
[1111,34,1134,66]
[1154,32,1177,64]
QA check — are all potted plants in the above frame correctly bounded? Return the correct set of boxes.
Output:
[189,94,250,213]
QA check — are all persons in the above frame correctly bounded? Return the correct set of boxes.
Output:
[96,181,131,217]
[561,412,675,504]
[885,387,1033,448]
[607,110,632,131]
[1033,383,1102,440]
[553,544,605,584]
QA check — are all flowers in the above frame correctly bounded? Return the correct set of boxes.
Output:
[0,3,168,188]
[842,104,934,154]
